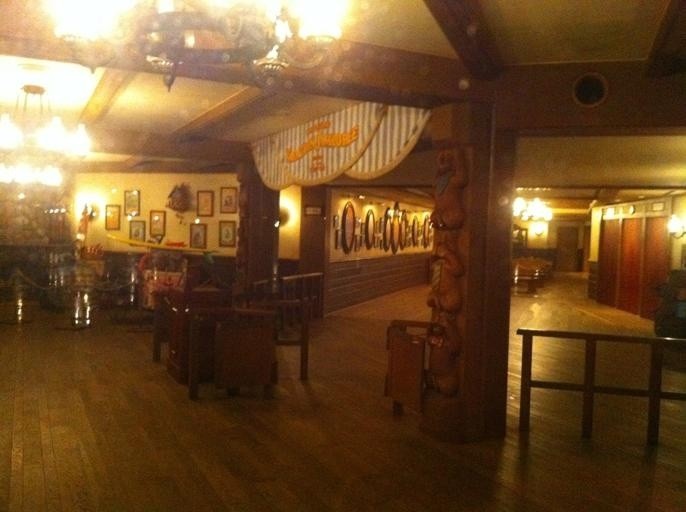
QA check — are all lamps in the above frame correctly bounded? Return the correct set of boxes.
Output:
[40,0,348,94]
[271,217,281,231]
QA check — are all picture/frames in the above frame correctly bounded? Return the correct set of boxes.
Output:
[380,219,384,230]
[129,221,145,246]
[364,209,375,249]
[373,236,379,245]
[197,191,213,217]
[124,192,138,217]
[189,223,207,247]
[356,218,361,236]
[413,216,419,246]
[334,215,340,228]
[342,201,356,255]
[361,224,366,234]
[375,222,379,232]
[399,209,407,249]
[220,221,236,247]
[355,238,362,250]
[363,237,366,244]
[422,216,430,249]
[105,205,120,230]
[392,201,400,254]
[335,230,344,248]
[221,188,236,213]
[384,207,391,250]
[150,211,164,237]
